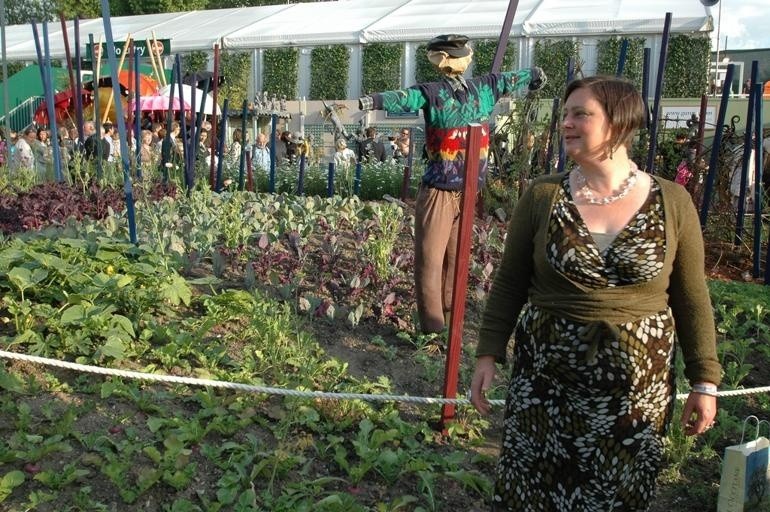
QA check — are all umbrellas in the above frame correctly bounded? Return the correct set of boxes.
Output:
[34,68,225,123]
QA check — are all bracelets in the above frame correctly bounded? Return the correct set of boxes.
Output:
[691,382,721,397]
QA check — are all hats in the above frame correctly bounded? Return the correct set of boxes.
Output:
[428,34,470,58]
[286,133,315,146]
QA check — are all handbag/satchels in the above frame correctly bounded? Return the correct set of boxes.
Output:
[714,415,769,511]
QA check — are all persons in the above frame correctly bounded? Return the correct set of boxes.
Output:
[0,121,122,183]
[332,125,429,169]
[322,35,549,332]
[513,131,546,170]
[659,124,769,208]
[471,73,725,510]
[129,121,313,177]
